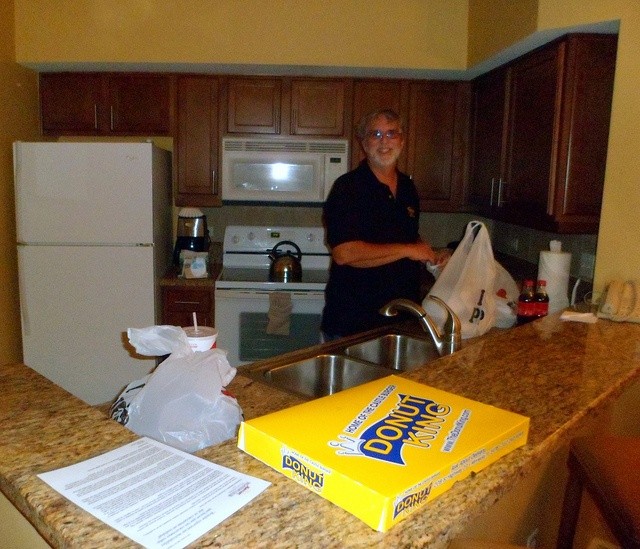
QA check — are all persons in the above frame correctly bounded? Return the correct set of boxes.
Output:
[319,108,437,344]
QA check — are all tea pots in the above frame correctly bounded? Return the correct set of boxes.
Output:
[268,241,304,282]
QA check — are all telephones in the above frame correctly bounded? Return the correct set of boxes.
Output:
[597,279,640,323]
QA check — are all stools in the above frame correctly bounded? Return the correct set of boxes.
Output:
[557,437,640,548]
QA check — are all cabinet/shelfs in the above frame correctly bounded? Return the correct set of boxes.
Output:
[40,71,174,135]
[222,76,353,138]
[163,283,215,329]
[469,33,620,234]
[175,72,222,208]
[352,80,469,214]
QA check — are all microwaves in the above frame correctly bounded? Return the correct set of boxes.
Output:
[220,137,349,202]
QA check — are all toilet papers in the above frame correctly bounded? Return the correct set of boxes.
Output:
[537,241,573,313]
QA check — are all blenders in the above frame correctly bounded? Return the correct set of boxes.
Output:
[174,207,211,269]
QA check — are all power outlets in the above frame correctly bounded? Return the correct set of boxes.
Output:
[208,226,214,236]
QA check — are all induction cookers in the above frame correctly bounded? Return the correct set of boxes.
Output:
[214,225,332,286]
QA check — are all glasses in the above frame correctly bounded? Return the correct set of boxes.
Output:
[362,129,403,139]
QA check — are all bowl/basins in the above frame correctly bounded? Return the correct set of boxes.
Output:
[179,325,217,355]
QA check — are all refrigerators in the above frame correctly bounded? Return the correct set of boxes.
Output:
[12,142,172,406]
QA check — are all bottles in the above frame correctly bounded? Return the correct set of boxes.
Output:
[517,279,534,326]
[534,279,549,321]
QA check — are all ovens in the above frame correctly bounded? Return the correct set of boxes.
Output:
[214,291,334,368]
